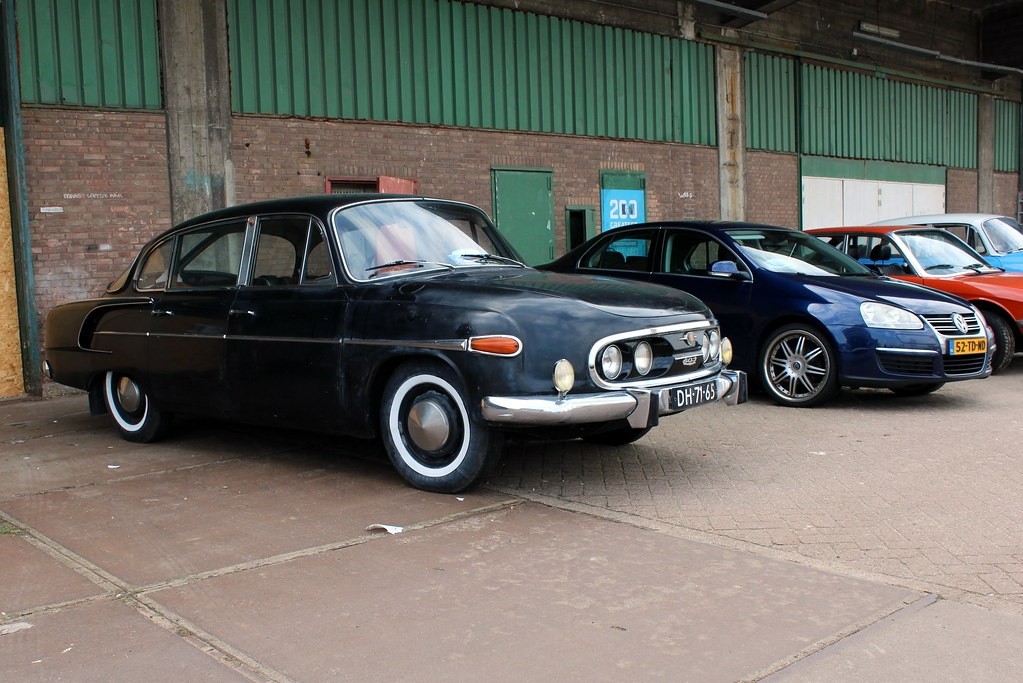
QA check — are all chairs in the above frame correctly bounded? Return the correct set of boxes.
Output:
[847,245,866,260]
[626,256,649,272]
[867,245,906,277]
[600,252,627,269]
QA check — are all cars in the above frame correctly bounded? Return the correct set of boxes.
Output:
[531,213,1023,408]
[43,193,749,495]
